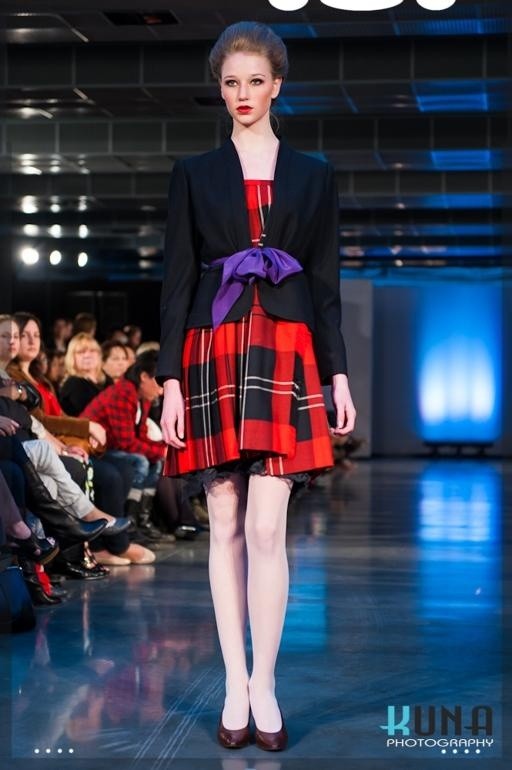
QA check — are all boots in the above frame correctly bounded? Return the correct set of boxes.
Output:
[4,458,108,545]
[126,493,209,550]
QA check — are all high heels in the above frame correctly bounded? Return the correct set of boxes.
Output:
[6,529,61,567]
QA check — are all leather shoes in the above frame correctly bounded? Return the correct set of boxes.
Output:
[253,705,286,750]
[17,545,156,605]
[219,703,250,749]
[102,517,132,536]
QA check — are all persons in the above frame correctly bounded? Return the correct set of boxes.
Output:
[152,18,360,754]
[0,312,366,633]
